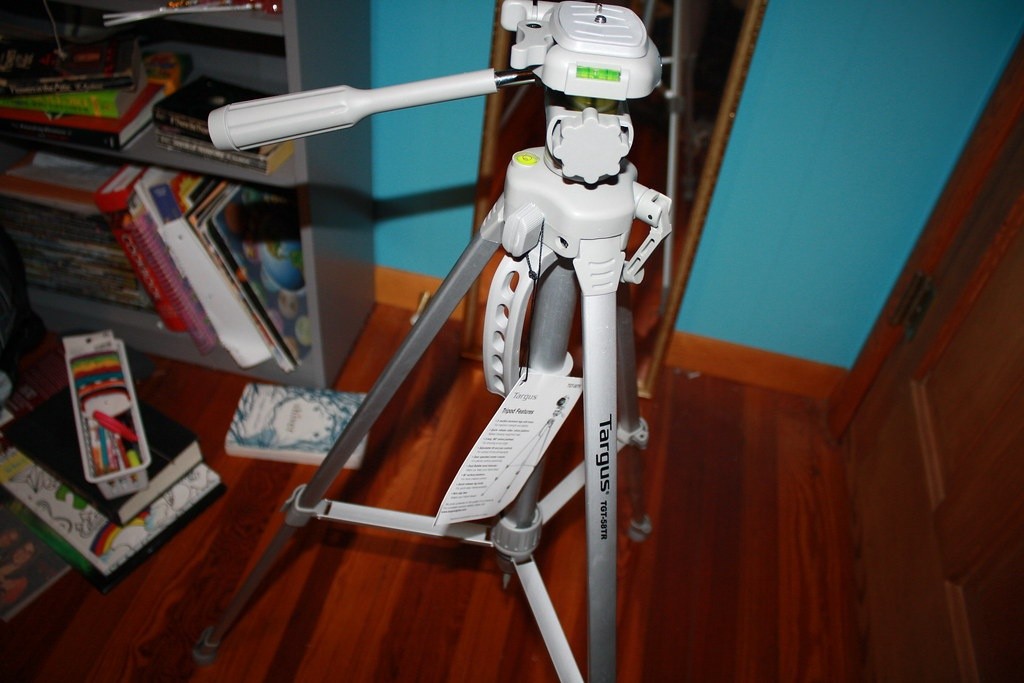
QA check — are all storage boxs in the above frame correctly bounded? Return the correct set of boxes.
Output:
[63,327,152,498]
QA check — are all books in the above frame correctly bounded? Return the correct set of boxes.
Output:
[0,381,225,595]
[0,23,313,374]
[222,374,374,471]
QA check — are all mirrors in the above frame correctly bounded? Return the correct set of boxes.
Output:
[464,1,769,400]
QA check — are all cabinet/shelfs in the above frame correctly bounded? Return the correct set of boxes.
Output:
[0,0,376,395]
